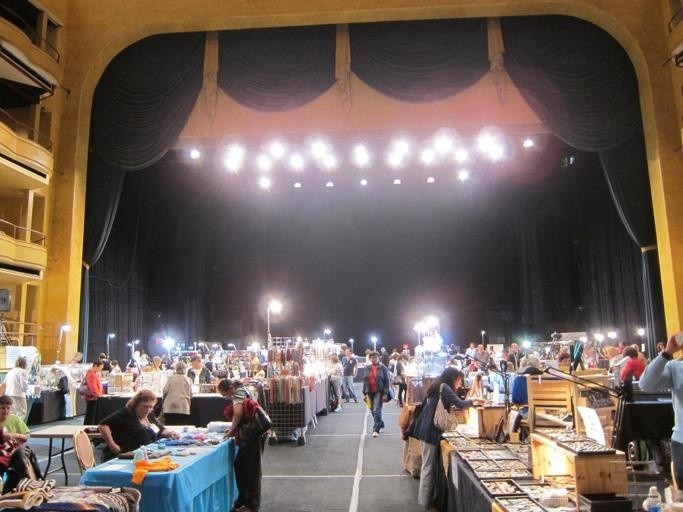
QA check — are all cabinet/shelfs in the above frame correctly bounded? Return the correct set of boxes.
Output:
[243,341,334,446]
[404,340,648,512]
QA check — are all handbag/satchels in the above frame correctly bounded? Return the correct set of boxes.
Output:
[433,382,458,433]
[79,370,94,396]
[384,391,392,401]
[238,386,272,443]
[407,396,427,440]
[56,371,68,397]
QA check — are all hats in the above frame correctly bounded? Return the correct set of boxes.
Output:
[517,357,549,375]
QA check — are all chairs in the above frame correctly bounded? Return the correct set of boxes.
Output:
[74,431,96,474]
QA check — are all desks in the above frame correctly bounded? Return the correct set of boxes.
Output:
[29,424,196,487]
[78,427,239,512]
[26,380,108,424]
[82,392,233,427]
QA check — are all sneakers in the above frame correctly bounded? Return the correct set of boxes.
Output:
[234,505,259,512]
[372,431,379,437]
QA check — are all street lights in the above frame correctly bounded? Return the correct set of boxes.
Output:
[211,343,223,350]
[126,339,138,358]
[228,343,236,350]
[197,343,208,350]
[106,334,115,356]
[348,338,354,349]
[266,300,281,349]
[371,336,377,351]
[55,325,70,364]
[481,331,486,346]
[323,329,331,341]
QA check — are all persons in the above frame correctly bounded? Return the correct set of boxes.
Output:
[639,334,683,492]
[410,368,485,509]
[218,379,269,510]
[364,346,389,435]
[384,333,664,477]
[0,396,42,496]
[4,358,28,419]
[71,341,264,426]
[98,391,178,463]
[50,366,76,419]
[328,345,356,412]
